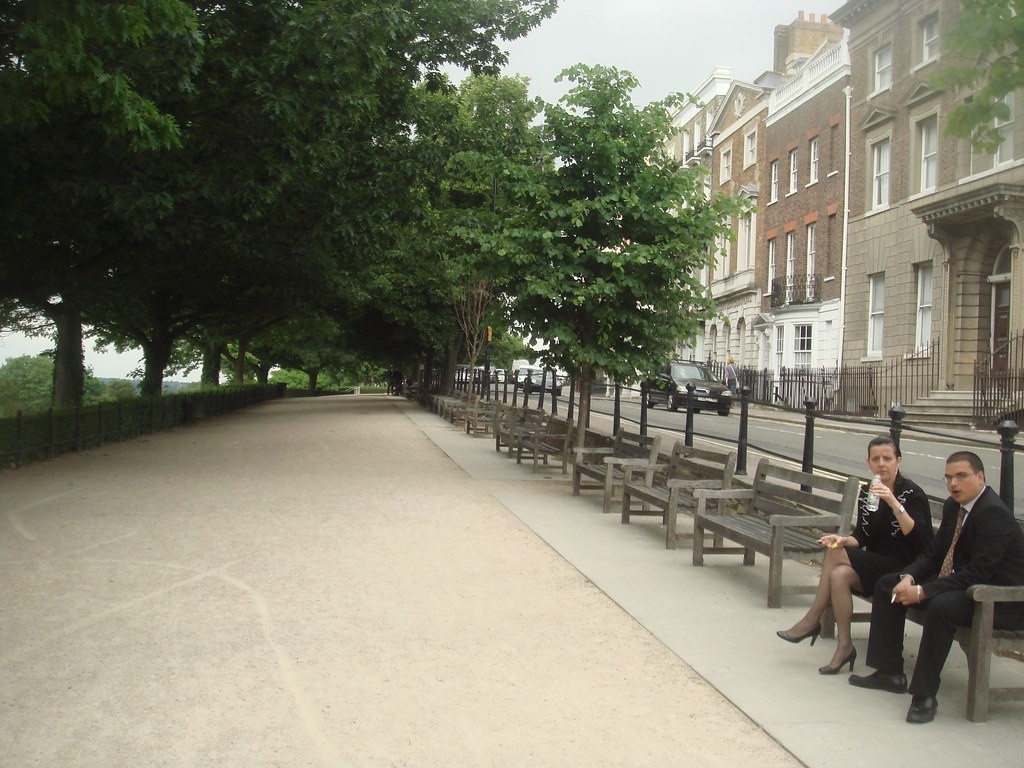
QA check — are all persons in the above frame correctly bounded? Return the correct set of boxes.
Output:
[725,357,738,409]
[848,450,1024,724]
[776,436,934,675]
[386,365,404,396]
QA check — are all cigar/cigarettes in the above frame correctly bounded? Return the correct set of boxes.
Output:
[890,594,896,604]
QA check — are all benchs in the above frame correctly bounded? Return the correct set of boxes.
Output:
[406,383,501,438]
[495,405,545,458]
[693,458,859,609]
[517,414,574,474]
[621,440,737,550]
[572,427,663,513]
[815,496,1024,722]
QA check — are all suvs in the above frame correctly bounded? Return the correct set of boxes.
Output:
[639,358,732,416]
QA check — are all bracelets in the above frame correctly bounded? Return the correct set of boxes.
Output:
[916,585,921,604]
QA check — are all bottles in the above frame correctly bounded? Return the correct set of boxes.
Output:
[866,474,882,512]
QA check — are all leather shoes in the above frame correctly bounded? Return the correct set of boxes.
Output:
[906,695,938,723]
[848,671,907,693]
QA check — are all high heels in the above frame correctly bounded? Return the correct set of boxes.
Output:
[777,622,821,646]
[819,646,857,673]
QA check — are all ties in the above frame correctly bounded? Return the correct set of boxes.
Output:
[937,508,968,579]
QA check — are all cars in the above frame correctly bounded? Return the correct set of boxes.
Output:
[516,365,542,389]
[523,368,562,397]
[453,363,512,385]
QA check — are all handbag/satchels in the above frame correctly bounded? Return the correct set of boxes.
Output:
[736,381,739,388]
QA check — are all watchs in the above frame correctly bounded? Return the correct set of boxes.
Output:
[892,506,905,515]
